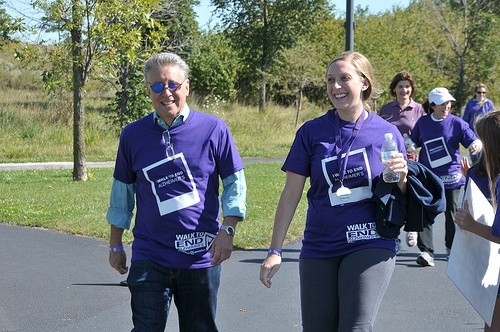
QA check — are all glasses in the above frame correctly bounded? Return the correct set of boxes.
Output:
[149,80,187,93]
[476,91,486,94]
[162,129,176,160]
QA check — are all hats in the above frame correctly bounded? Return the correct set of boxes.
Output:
[428,87,456,106]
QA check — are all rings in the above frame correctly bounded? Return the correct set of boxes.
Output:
[404,163,406,167]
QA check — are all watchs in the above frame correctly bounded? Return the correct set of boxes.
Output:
[221,226,235,236]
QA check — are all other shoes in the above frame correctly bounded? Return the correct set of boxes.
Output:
[396,239,401,255]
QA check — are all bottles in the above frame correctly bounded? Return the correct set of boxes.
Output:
[403,134,417,157]
[380,133,401,183]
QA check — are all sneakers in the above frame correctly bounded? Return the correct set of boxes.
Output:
[406,232,419,247]
[417,251,435,267]
[445,248,450,259]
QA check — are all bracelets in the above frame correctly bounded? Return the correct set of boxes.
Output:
[268,248,282,256]
[109,246,123,252]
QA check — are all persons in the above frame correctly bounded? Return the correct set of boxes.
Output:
[410,87,482,266]
[379,71,427,252]
[455,110,500,332]
[463,84,494,164]
[106,53,247,332]
[260,51,408,332]
